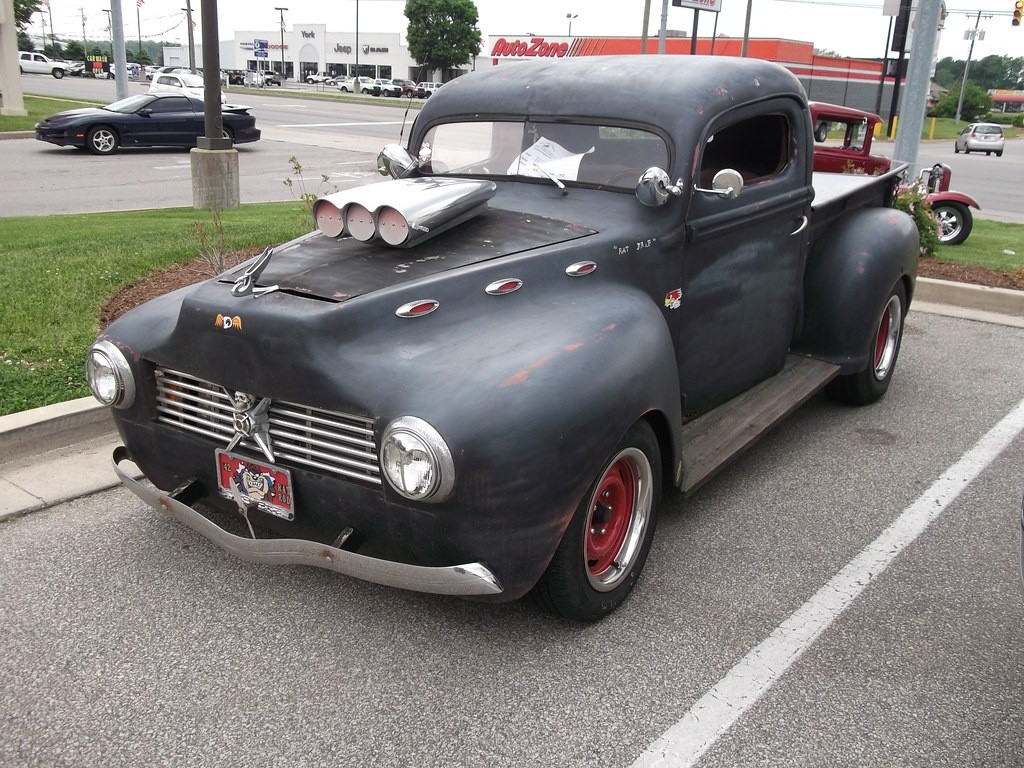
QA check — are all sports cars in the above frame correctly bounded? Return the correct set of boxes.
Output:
[36,92,261,156]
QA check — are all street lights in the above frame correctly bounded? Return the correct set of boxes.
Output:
[274,7,290,86]
[103,8,114,63]
[565,13,578,37]
[181,7,195,69]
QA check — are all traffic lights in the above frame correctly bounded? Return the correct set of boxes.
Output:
[1012,0,1024,26]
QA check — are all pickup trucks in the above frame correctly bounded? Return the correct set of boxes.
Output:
[337,77,378,95]
[105,53,920,624]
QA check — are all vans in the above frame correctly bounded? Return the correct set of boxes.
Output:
[147,73,227,105]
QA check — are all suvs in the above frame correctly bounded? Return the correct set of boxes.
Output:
[804,99,984,248]
[306,71,333,85]
[392,79,418,99]
[18,51,72,81]
[417,82,444,100]
[370,79,403,98]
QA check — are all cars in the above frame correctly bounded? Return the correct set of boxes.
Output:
[325,76,352,86]
[48,57,287,88]
[814,118,833,143]
[954,123,1006,157]
